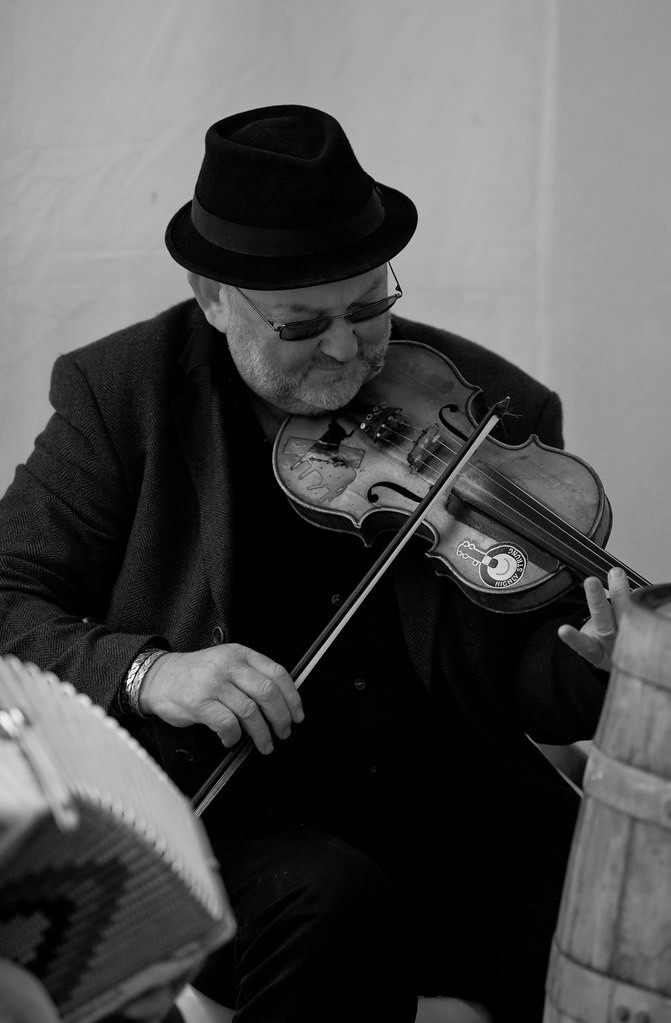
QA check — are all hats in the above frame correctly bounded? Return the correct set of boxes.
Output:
[165,104,419,292]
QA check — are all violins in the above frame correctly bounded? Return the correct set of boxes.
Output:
[271,339,655,615]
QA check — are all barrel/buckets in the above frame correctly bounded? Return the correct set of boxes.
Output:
[542,582,671,1023]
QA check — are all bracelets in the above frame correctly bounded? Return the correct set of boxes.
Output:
[115,645,168,718]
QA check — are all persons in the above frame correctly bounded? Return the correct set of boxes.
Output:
[0,105,671,1023]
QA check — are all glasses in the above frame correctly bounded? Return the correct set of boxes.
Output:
[236,261,403,342]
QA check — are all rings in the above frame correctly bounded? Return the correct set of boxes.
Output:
[593,626,616,642]
